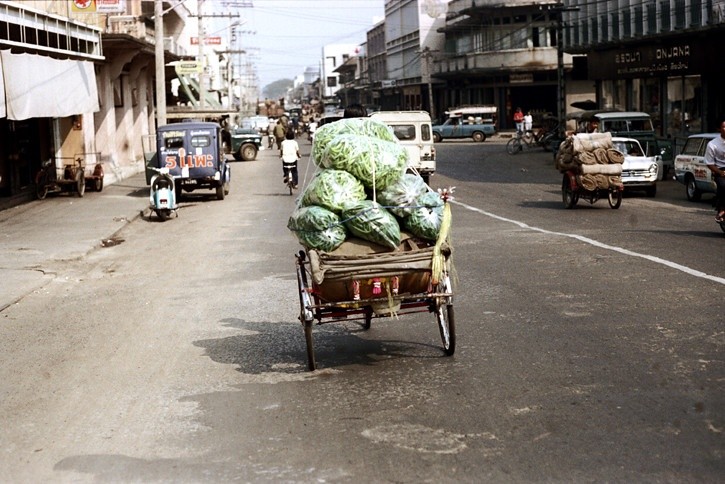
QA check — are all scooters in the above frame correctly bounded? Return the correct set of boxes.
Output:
[147,166,179,222]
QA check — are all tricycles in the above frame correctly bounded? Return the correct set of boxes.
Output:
[561,170,625,209]
[294,245,456,370]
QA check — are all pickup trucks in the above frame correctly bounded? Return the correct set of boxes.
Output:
[432,116,496,142]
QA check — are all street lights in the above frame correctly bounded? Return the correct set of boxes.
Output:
[196,18,248,110]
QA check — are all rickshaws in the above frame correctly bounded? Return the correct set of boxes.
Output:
[506,117,561,154]
[31,154,105,200]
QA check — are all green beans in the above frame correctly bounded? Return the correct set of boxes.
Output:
[286,120,444,252]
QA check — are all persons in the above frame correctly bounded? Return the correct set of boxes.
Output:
[468,116,483,125]
[704,121,725,221]
[266,111,318,150]
[280,131,301,189]
[591,121,598,133]
[514,107,532,137]
[343,104,368,119]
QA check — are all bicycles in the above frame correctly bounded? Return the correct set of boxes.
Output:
[280,155,301,195]
[268,136,275,149]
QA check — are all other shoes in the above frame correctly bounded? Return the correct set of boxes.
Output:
[284,178,287,183]
[294,185,298,189]
[715,211,725,221]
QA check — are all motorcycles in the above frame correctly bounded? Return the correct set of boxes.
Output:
[157,119,230,200]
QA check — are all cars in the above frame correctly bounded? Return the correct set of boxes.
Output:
[371,110,437,185]
[674,133,718,201]
[611,138,660,197]
